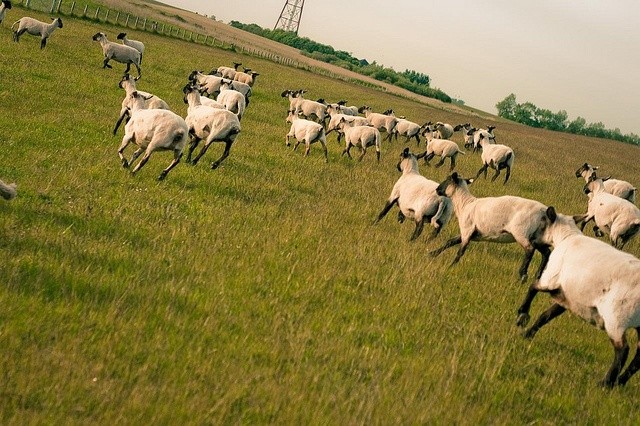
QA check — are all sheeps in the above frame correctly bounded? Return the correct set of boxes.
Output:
[422,123,435,146]
[469,133,514,185]
[325,103,370,147]
[434,121,454,140]
[117,91,189,180]
[215,79,247,121]
[217,62,243,79]
[374,146,452,243]
[222,67,251,82]
[358,104,399,143]
[463,123,473,130]
[415,127,465,172]
[382,109,423,148]
[117,32,144,65]
[183,78,228,144]
[484,125,496,143]
[208,68,253,108]
[91,32,142,81]
[280,89,299,118]
[0,0,11,24]
[11,17,63,50]
[285,110,328,163]
[427,122,441,139]
[467,128,489,152]
[571,170,640,251]
[293,86,328,131]
[428,172,551,283]
[189,69,222,98]
[114,72,169,135]
[181,82,241,169]
[336,99,359,117]
[514,206,640,390]
[234,70,261,87]
[454,124,474,149]
[315,96,340,124]
[574,162,638,236]
